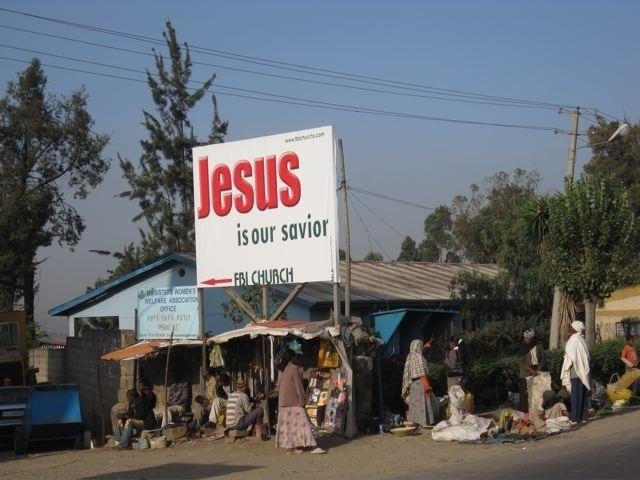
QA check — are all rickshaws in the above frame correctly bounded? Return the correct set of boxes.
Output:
[13,382,93,455]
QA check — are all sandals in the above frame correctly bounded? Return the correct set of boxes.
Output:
[112,444,134,451]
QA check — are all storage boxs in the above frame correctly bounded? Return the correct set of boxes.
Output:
[306,404,325,418]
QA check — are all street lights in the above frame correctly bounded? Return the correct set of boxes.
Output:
[547,118,632,352]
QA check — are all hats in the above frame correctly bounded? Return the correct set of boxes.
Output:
[235,379,247,392]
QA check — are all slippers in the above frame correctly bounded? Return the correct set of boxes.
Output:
[309,447,327,454]
[285,449,304,456]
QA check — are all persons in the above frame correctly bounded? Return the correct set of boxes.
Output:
[444,336,466,391]
[560,321,591,420]
[274,349,326,455]
[620,334,640,399]
[401,336,440,429]
[113,365,268,451]
[518,328,541,413]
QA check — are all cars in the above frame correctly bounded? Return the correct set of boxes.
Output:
[0,385,36,427]
